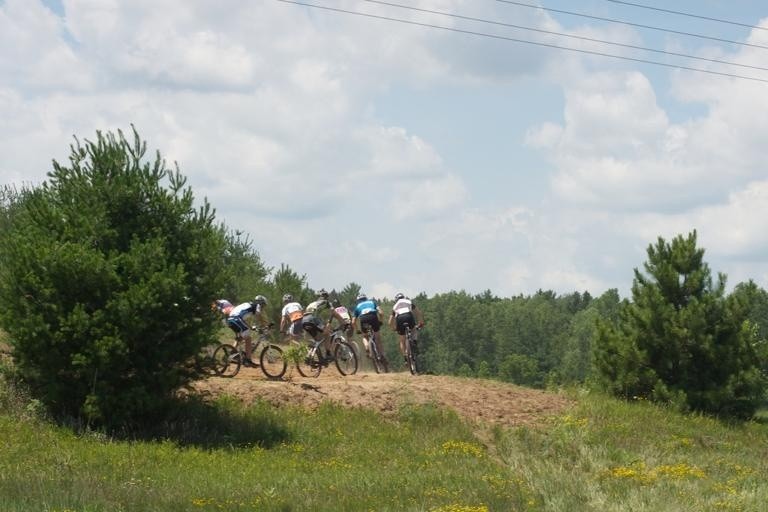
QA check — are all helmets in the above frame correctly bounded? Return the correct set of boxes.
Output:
[357,294,366,300]
[255,295,268,305]
[396,293,404,300]
[283,294,293,302]
[319,292,340,307]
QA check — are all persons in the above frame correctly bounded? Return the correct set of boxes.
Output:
[351,293,387,364]
[227,295,272,367]
[216,299,233,328]
[278,292,352,367]
[388,293,425,363]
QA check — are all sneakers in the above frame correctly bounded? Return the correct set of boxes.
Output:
[245,359,261,368]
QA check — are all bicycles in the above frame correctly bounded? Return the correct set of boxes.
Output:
[183,321,424,379]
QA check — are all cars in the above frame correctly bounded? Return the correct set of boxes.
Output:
[216,299,235,319]
